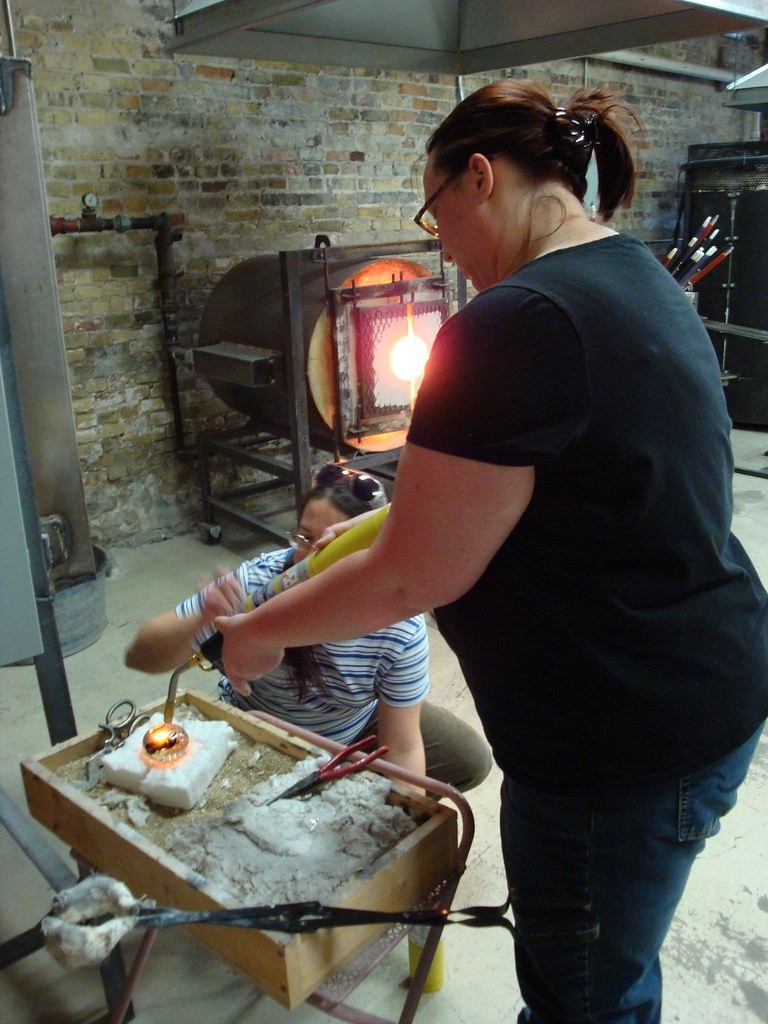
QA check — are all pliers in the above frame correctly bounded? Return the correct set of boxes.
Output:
[266,735,389,809]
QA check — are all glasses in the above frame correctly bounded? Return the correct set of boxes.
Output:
[414,154,495,238]
[312,462,384,506]
[286,530,318,558]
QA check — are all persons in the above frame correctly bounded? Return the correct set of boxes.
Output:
[213,79,768,1023]
[121,461,429,780]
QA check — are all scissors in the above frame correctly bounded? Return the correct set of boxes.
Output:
[74,697,151,793]
